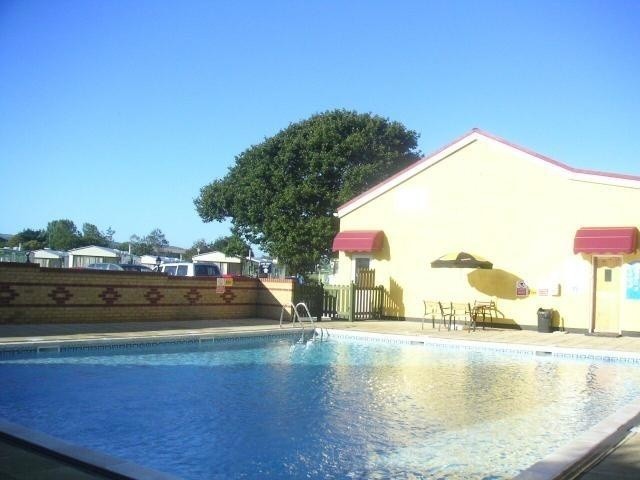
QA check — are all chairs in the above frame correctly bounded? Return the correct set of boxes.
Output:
[422,298,493,332]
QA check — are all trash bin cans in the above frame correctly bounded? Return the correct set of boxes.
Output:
[538,308,552,332]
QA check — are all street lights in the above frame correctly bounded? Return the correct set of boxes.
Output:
[249,232,265,277]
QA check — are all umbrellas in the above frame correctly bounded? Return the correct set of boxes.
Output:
[429,248,496,271]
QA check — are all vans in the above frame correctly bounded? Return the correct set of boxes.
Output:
[159,263,221,277]
[88,263,154,273]
